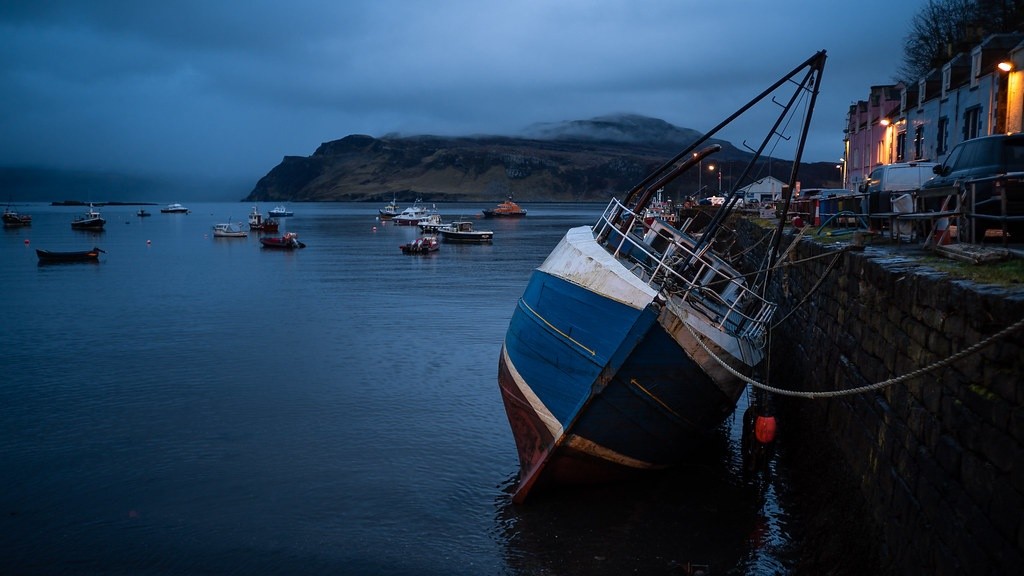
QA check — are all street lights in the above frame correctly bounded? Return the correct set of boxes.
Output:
[693,152,702,200]
[836,164,843,177]
[997,60,1015,134]
[879,118,894,164]
[840,158,847,189]
[708,162,723,198]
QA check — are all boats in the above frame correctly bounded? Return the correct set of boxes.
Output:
[496,48,829,510]
[246,206,281,234]
[135,208,151,218]
[914,132,1024,246]
[481,192,528,218]
[268,205,295,218]
[399,234,442,255]
[160,202,189,213]
[261,233,307,251]
[1,195,33,228]
[34,245,109,262]
[70,202,106,230]
[378,192,495,242]
[210,216,248,239]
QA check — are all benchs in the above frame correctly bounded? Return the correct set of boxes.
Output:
[867,186,964,248]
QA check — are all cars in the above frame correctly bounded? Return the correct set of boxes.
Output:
[791,187,856,200]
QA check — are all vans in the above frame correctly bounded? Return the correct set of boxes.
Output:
[858,160,942,228]
